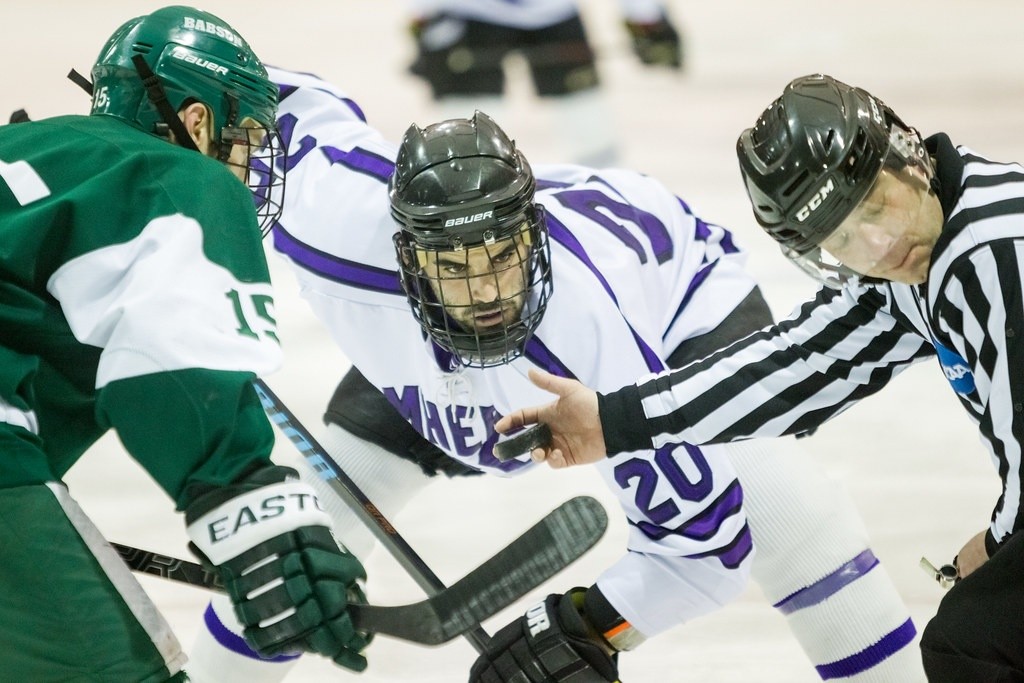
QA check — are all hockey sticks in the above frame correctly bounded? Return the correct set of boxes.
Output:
[108,382,608,648]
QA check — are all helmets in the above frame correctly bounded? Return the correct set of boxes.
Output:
[388,110,553,369]
[737,73,913,270]
[67,5,285,238]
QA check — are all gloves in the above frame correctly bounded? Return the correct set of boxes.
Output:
[186,465,374,672]
[468,587,622,683]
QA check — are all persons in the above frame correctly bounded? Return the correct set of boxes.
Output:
[0,0,375,683]
[494,70,1024,683]
[407,0,688,101]
[242,64,931,683]
